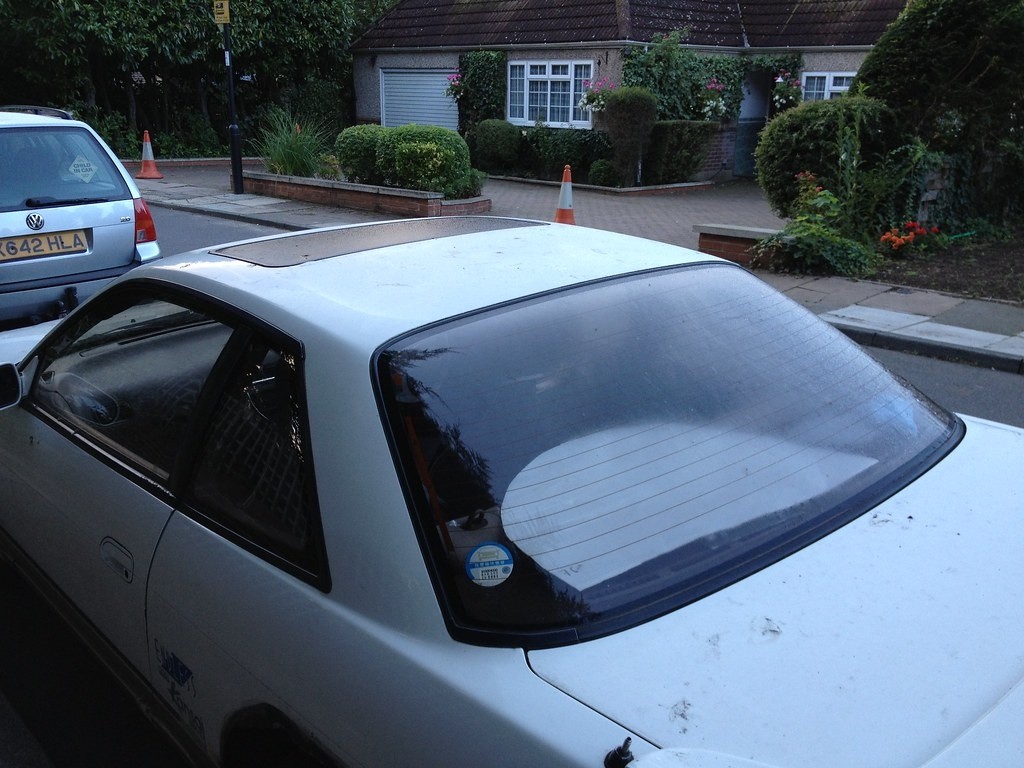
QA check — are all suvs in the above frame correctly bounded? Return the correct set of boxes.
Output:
[1,103,161,331]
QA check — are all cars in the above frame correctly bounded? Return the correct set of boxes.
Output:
[0,216,1024,768]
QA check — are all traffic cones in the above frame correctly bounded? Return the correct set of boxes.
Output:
[134,130,165,181]
[553,166,578,226]
